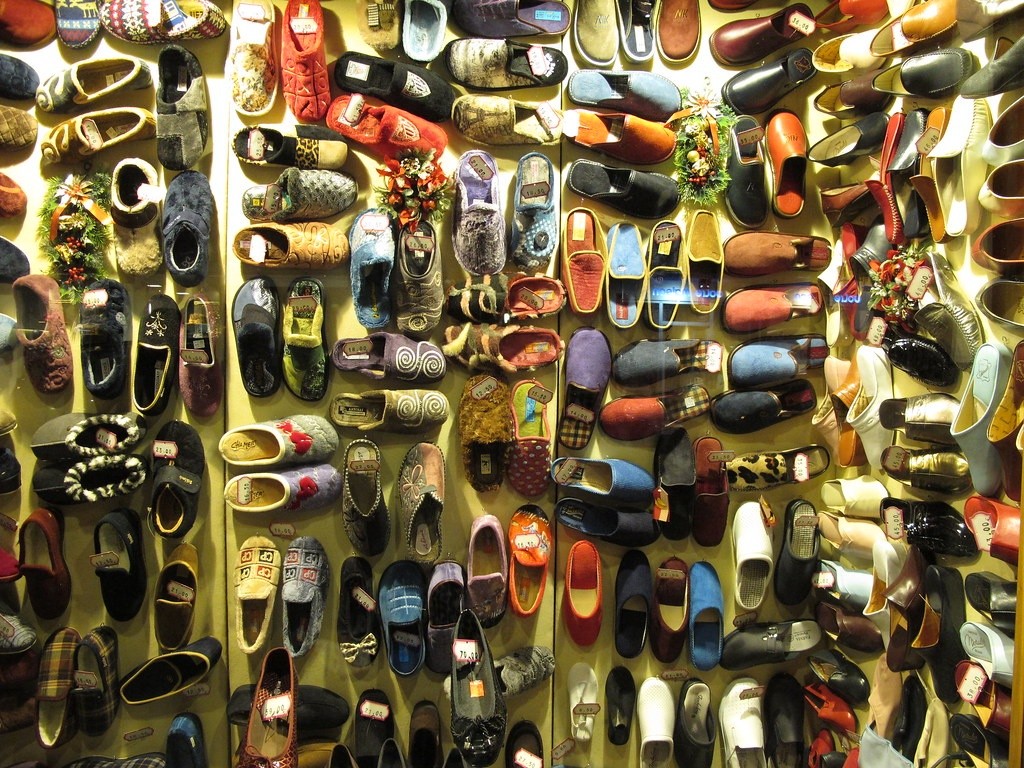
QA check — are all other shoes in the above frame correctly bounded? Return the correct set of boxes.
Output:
[0,0,1024,768]
